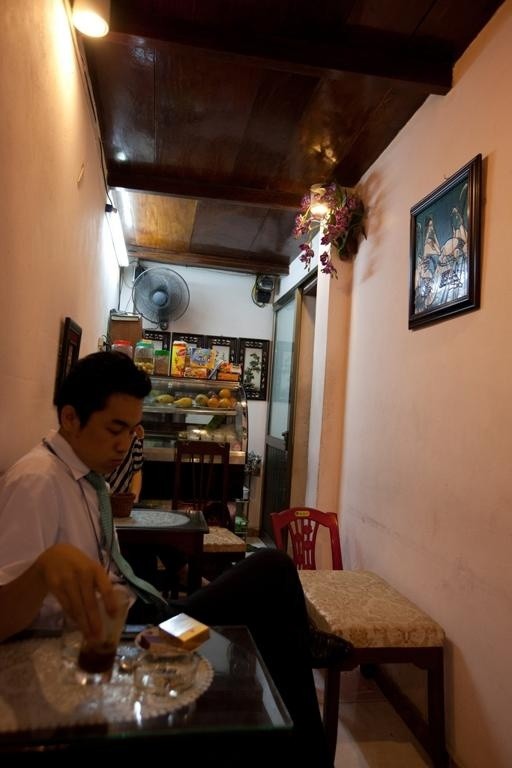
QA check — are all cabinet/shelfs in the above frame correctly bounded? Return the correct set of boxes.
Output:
[136,373,250,510]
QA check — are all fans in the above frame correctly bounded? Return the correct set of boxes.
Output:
[130,266,192,330]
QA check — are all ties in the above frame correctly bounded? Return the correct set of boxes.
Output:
[85,470,168,608]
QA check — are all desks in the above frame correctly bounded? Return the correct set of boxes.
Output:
[106,508,209,602]
[1,624,294,767]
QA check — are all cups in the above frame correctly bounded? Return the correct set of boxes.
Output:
[110,492,136,518]
[74,588,132,686]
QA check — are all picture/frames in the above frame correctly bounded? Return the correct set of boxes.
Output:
[407,150,482,331]
[53,317,83,404]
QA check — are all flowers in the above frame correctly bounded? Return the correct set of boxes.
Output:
[291,176,369,280]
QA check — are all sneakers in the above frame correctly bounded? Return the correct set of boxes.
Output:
[313,628,354,670]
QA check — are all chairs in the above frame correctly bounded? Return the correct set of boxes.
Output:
[171,440,238,532]
[267,506,343,571]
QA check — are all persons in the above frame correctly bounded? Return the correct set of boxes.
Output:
[1,348,365,765]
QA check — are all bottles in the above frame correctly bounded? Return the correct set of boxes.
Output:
[152,350,171,377]
[134,341,155,376]
[111,339,133,360]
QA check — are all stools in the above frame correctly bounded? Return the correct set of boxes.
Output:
[202,525,247,579]
[296,570,445,768]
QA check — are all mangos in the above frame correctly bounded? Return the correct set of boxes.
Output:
[174,398,192,408]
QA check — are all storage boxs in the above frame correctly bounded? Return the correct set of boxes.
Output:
[111,317,142,348]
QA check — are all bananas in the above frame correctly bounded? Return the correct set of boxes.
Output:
[154,395,174,403]
